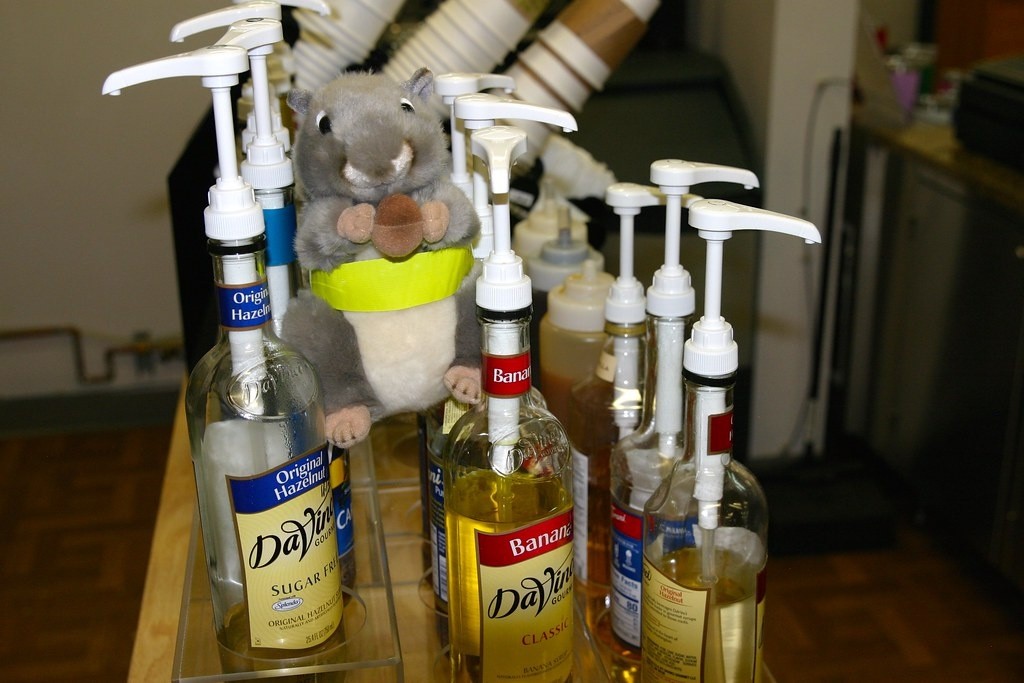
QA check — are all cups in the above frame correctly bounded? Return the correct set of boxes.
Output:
[292,0,660,182]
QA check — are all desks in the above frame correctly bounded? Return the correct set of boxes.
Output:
[122,371,781,683]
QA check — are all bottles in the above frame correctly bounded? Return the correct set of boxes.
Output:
[531,206,604,392]
[512,179,587,278]
[540,260,615,430]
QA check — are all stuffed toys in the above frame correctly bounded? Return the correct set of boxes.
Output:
[277,68,486,449]
